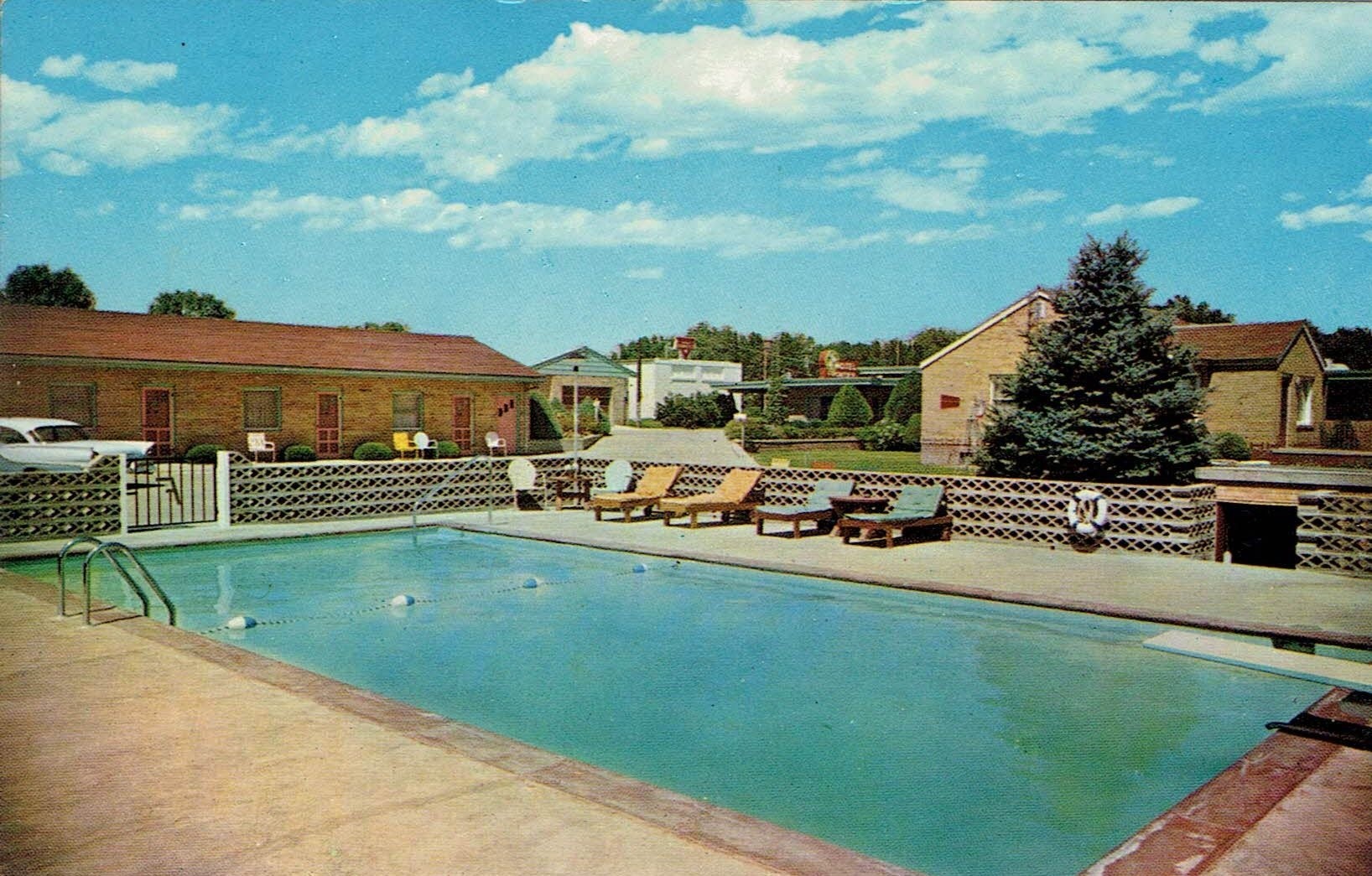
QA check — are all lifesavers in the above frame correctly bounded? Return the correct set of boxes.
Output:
[1067,489,1107,536]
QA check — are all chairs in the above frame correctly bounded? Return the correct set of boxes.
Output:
[659,470,765,528]
[755,480,856,539]
[589,460,634,509]
[393,431,418,459]
[508,459,547,510]
[414,432,439,458]
[247,433,276,463]
[484,431,507,457]
[838,483,954,548]
[593,464,684,522]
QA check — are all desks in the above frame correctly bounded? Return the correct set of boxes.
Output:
[549,475,596,510]
[830,496,891,538]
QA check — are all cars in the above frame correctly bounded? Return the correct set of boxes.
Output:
[0,416,156,473]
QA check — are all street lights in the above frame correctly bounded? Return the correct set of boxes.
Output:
[573,366,579,460]
